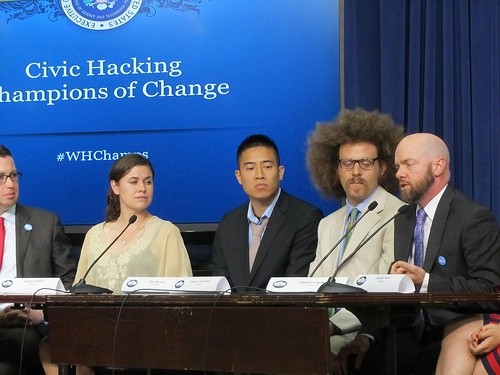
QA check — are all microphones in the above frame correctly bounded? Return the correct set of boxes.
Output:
[70,214,138,293]
[309,201,378,277]
[318,205,408,293]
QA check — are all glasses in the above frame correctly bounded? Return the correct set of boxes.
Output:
[338,156,379,170]
[0,171,22,184]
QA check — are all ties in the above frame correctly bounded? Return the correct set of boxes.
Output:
[414,208,428,293]
[340,208,360,262]
[0,217,5,272]
[248,217,269,274]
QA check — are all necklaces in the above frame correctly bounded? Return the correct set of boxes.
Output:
[118,213,147,245]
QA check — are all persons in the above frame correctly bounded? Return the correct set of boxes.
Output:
[212,135,325,293]
[334,132,500,375]
[40,154,193,375]
[307,107,410,375]
[0,144,77,375]
[435,313,500,375]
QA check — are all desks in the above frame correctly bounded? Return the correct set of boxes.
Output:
[0,291,500,375]
